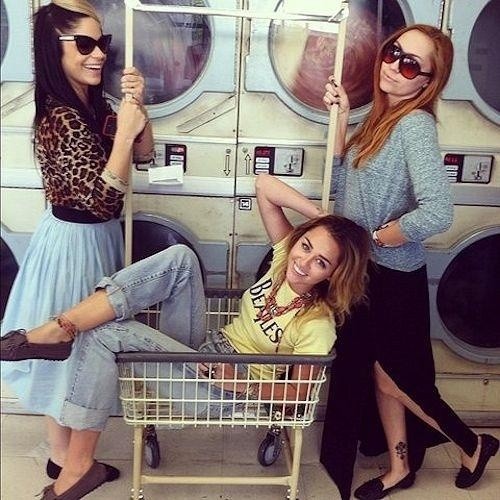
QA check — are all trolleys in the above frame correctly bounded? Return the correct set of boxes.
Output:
[115,286,339,500]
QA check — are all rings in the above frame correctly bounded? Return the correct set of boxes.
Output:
[204,368,216,380]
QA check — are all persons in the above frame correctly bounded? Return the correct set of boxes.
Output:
[321,24,500,499]
[1,1,158,499]
[2,172,373,499]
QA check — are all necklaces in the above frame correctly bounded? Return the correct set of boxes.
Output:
[257,266,317,322]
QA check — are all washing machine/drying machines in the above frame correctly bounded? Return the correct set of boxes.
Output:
[0,1,500,428]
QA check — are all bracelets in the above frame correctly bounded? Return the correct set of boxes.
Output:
[243,380,258,400]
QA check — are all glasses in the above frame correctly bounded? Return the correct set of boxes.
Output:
[58,33,112,55]
[381,43,433,80]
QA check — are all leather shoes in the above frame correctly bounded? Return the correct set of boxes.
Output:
[0,328,74,361]
[35,459,108,500]
[455,433,499,488]
[355,470,415,500]
[47,458,120,482]
